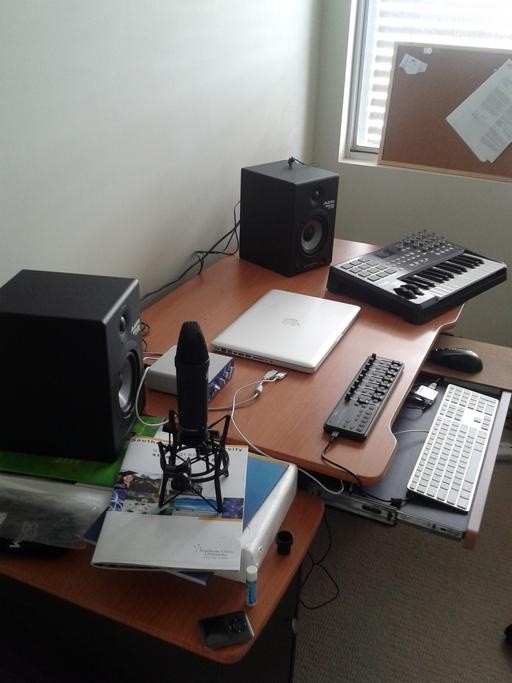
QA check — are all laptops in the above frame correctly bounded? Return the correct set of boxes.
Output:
[211,289,361,374]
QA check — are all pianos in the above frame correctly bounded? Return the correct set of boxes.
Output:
[327,231,508,323]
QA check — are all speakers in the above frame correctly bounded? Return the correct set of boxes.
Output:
[239,160,339,277]
[0,269,146,463]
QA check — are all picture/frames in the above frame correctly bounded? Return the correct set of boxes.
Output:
[377,42,512,183]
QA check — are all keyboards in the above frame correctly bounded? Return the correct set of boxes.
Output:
[407,383,499,512]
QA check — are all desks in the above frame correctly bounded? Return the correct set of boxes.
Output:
[1,236,511,683]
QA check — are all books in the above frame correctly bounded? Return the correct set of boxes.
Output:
[0,413,291,589]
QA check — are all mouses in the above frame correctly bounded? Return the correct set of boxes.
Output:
[430,347,483,373]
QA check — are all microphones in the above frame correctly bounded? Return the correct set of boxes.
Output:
[174,320,210,446]
